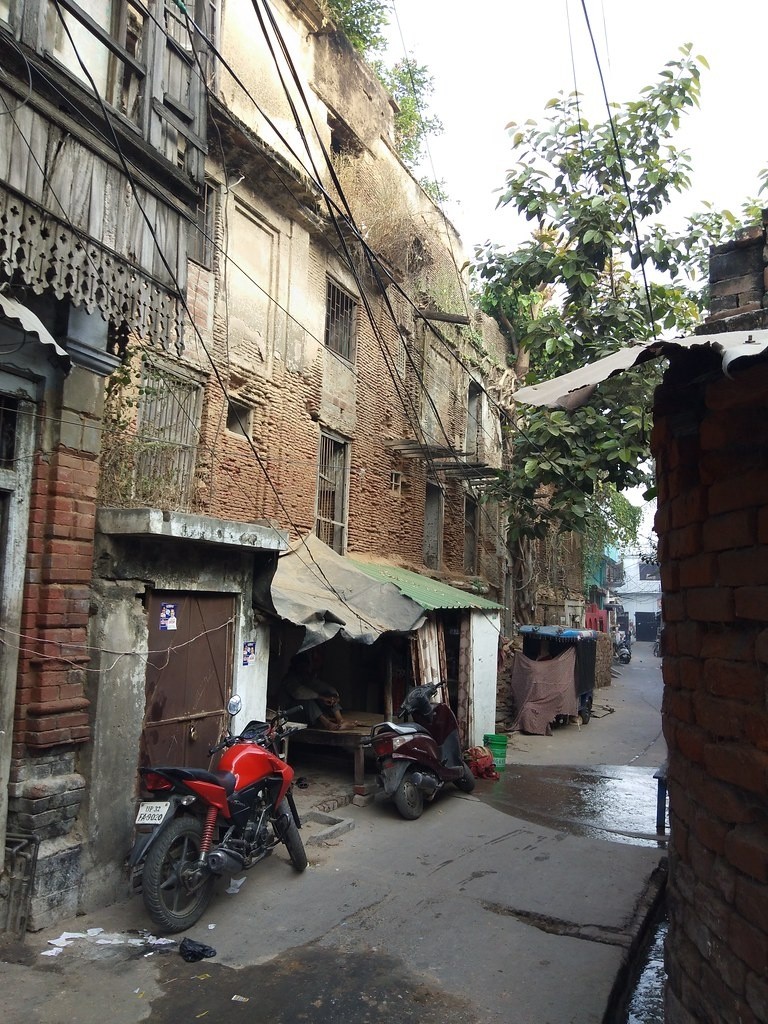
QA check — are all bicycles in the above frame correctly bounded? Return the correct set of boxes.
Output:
[653,639,661,657]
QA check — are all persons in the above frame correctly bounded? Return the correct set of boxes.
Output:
[625,627,631,641]
[278,655,359,731]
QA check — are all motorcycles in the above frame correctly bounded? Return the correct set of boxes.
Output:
[128,694,307,933]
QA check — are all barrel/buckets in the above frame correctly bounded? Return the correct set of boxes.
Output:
[483,734,508,772]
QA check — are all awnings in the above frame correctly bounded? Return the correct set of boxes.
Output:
[516,329,768,409]
[0,292,76,378]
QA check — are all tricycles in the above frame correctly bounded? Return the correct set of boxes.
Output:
[519,626,597,735]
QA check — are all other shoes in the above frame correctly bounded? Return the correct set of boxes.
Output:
[297,775,309,788]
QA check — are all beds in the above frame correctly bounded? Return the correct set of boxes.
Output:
[289,711,398,785]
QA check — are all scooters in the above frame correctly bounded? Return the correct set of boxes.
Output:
[365,680,475,820]
[613,640,632,664]
